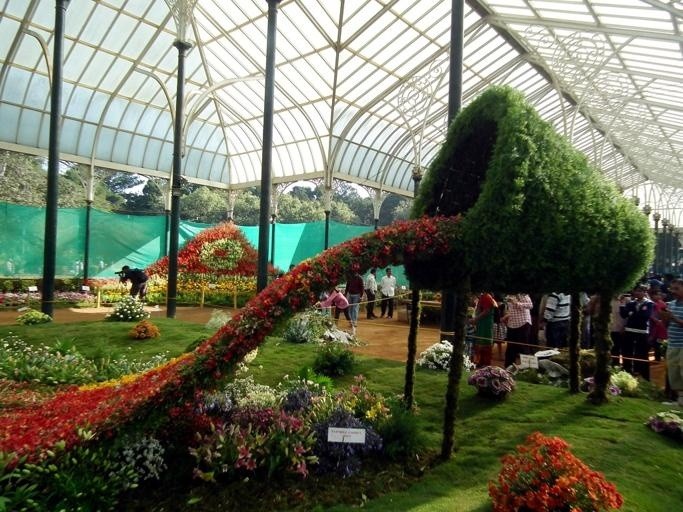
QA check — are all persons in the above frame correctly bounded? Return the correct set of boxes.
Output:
[318,292,332,326]
[468,272,681,408]
[343,275,364,327]
[379,268,397,319]
[117,265,149,299]
[322,286,353,331]
[289,264,295,271]
[363,268,379,321]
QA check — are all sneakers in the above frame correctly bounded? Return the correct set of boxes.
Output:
[365,310,394,321]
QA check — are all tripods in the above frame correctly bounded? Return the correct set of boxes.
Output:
[106,276,130,317]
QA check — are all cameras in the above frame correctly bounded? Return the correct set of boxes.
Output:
[115,271,124,277]
